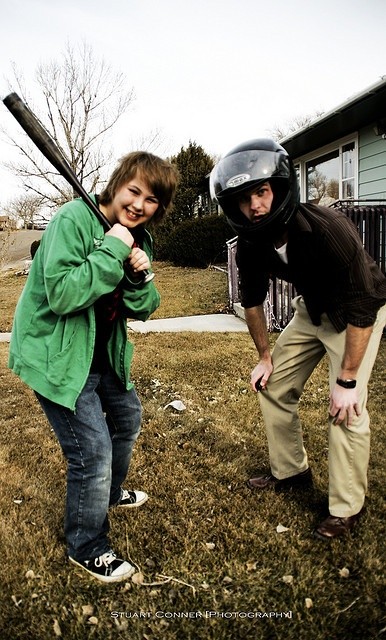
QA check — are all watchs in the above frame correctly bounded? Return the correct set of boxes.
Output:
[336,377,357,389]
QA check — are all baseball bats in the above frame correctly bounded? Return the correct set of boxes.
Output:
[3,92,155,283]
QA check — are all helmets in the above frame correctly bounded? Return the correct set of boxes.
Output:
[209,136,301,247]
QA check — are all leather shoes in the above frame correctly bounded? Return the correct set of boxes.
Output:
[246,467,314,492]
[314,496,366,540]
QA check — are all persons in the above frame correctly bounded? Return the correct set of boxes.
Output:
[7,151,181,584]
[208,138,386,541]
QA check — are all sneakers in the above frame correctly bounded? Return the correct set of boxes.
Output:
[67,547,135,584]
[116,488,149,508]
